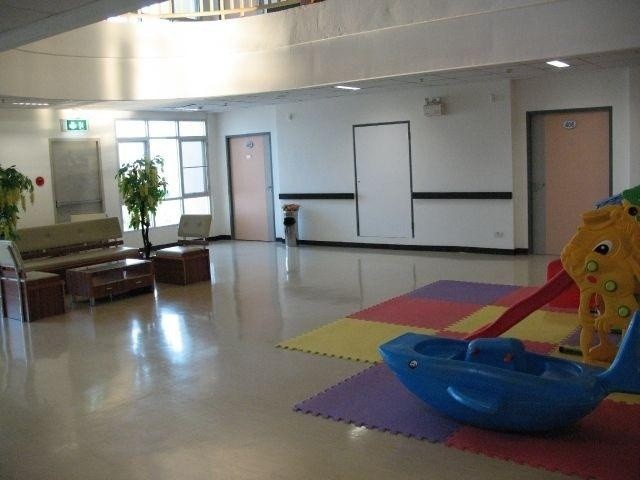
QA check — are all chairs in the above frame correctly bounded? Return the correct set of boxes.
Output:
[2,325,69,404]
[0,215,213,322]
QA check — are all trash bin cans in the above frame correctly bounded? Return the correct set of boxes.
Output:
[283,211,300,247]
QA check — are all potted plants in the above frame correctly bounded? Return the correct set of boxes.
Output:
[114,155,169,274]
